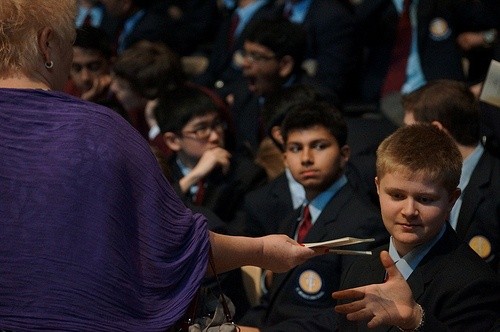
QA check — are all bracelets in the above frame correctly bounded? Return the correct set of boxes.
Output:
[399,303,424,332]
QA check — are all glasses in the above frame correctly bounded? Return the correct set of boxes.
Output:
[180,119,223,138]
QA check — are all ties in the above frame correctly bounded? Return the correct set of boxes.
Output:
[297,206,312,244]
[195,180,205,205]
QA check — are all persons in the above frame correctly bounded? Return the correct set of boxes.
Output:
[68,0,500,236]
[233,123,489,332]
[400,78,500,271]
[238,101,385,326]
[0,0,327,332]
[153,85,270,225]
[332,251,500,332]
[151,145,249,324]
